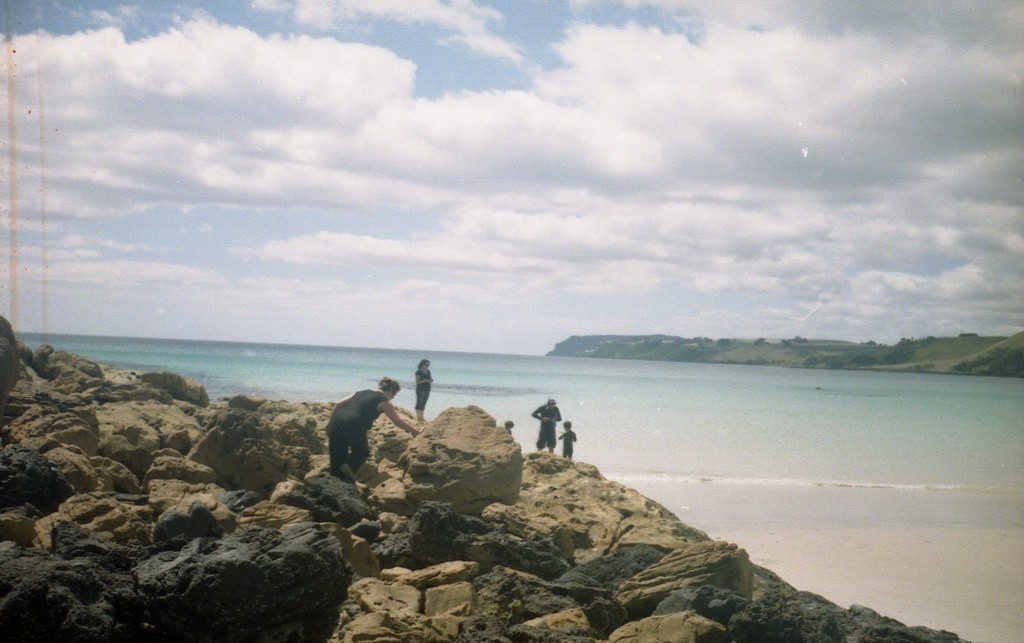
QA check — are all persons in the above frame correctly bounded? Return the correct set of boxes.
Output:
[559,421,576,458]
[415,359,433,422]
[327,377,427,479]
[532,398,561,452]
[505,421,514,434]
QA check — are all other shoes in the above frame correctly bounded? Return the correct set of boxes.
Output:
[341,464,354,479]
